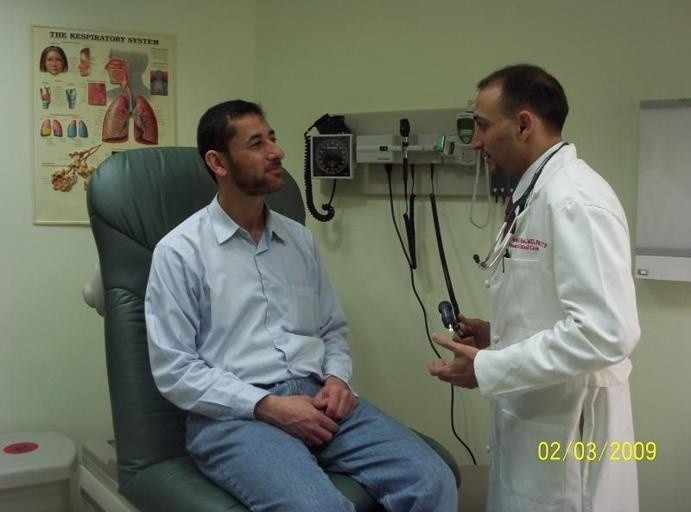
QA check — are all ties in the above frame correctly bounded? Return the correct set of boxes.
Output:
[505,198,514,231]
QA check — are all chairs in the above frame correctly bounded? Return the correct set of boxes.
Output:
[76,139,466,510]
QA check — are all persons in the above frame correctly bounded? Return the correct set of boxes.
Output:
[144,99,458,512]
[40,46,68,74]
[78,47,91,76]
[426,64,641,512]
